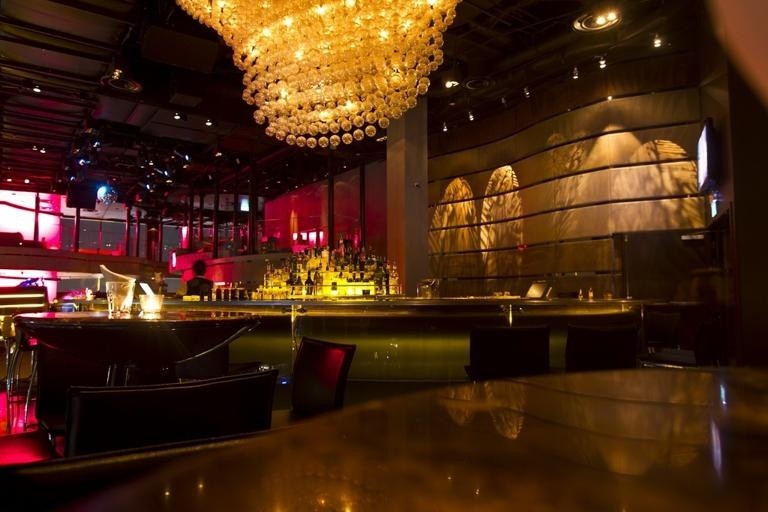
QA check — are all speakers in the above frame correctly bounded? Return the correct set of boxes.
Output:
[66,184,97,210]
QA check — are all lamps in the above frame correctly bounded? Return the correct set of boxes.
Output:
[175,0,464,148]
[565,0,658,34]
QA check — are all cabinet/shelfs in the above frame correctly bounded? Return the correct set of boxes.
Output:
[251,239,405,301]
[0,246,158,301]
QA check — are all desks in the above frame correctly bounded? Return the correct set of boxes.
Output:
[13,301,256,422]
[73,367,767,512]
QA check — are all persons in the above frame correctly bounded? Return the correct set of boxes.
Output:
[176,260,216,298]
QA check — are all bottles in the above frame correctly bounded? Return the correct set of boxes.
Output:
[265,244,390,297]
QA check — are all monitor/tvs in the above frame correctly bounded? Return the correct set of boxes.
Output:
[694,118,721,196]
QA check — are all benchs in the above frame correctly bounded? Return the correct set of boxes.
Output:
[0,287,49,315]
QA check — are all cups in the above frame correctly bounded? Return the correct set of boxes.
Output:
[137,294,165,313]
[106,280,135,319]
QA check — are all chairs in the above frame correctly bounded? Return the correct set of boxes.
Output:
[465,304,728,383]
[2,317,37,431]
[52,369,278,458]
[272,337,356,428]
